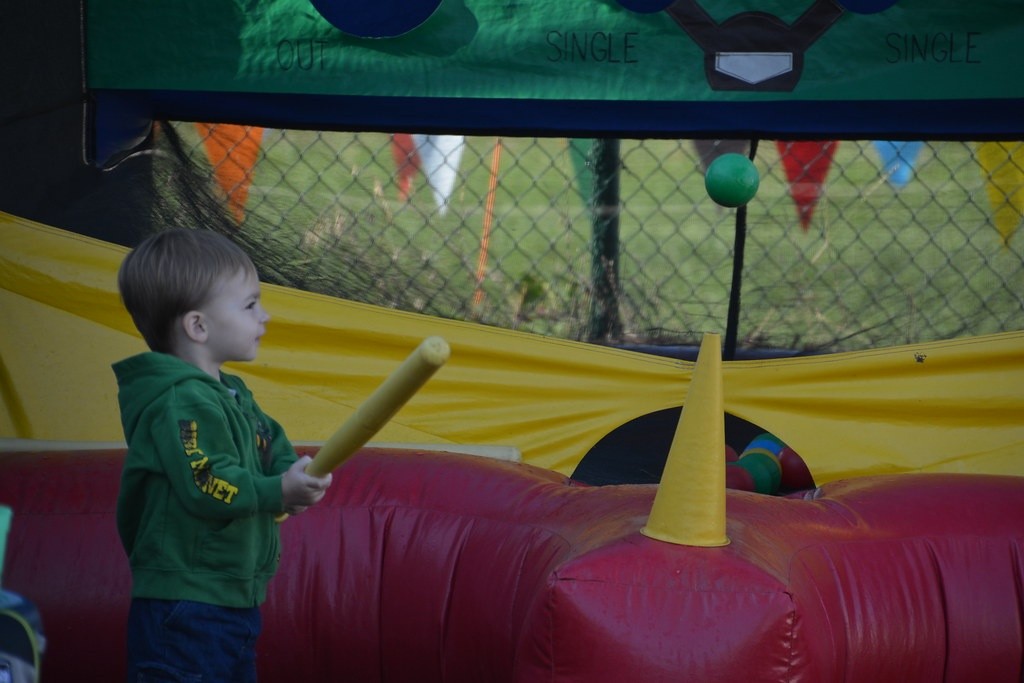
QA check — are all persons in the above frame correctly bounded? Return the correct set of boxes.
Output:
[112,229,331,683]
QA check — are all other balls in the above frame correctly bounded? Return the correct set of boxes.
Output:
[701,150,763,210]
[722,429,821,496]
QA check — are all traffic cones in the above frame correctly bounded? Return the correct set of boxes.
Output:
[631,334,737,548]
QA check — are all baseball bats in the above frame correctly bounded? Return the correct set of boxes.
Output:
[271,332,452,528]
